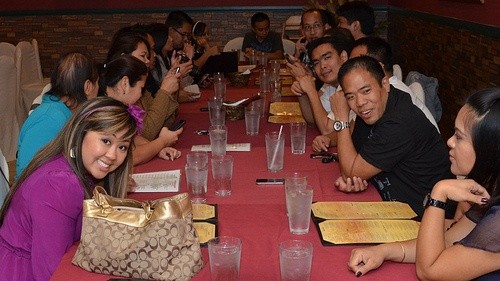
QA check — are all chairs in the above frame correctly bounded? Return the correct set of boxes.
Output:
[223,37,244,53]
[282,16,305,41]
[0,39,51,208]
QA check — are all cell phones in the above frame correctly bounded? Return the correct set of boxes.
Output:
[256,178,283,184]
[171,120,187,131]
[175,67,180,74]
[285,54,295,65]
[311,153,333,158]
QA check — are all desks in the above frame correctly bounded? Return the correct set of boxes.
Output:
[49,59,421,281]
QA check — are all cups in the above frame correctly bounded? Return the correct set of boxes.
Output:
[184,72,234,204]
[265,131,284,171]
[291,122,306,155]
[207,235,242,281]
[278,239,313,281]
[244,106,261,135]
[284,172,313,235]
[248,48,282,117]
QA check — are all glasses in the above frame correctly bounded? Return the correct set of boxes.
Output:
[173,28,192,40]
[302,24,323,30]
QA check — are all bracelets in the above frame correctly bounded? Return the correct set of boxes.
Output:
[396,241,405,263]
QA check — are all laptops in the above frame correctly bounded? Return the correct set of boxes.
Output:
[196,52,239,76]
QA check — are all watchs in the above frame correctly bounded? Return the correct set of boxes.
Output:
[333,120,350,132]
[423,193,448,211]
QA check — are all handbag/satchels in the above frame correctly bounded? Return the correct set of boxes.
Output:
[71,186,205,281]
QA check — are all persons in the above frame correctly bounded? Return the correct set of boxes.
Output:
[348,84,500,281]
[15,4,458,220]
[0,96,137,281]
